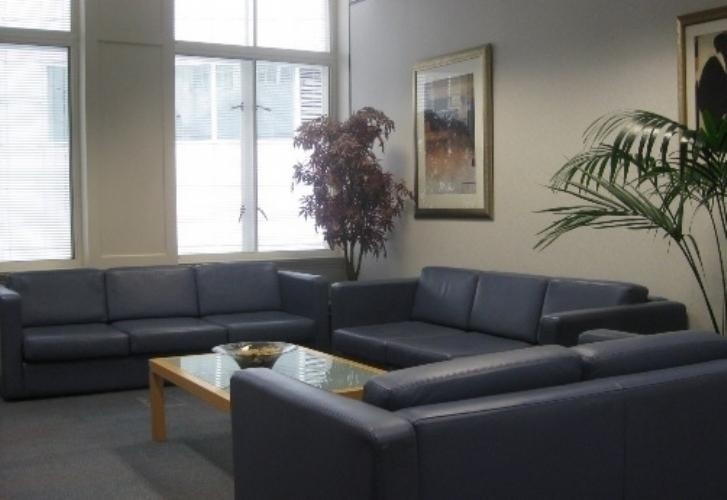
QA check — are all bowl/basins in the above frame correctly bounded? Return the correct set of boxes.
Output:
[209,339,298,370]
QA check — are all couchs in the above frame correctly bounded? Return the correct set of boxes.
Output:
[230,329,727,498]
[329,261,689,375]
[1,258,333,401]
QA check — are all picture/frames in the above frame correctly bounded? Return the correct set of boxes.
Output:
[410,40,494,223]
[676,5,726,186]
